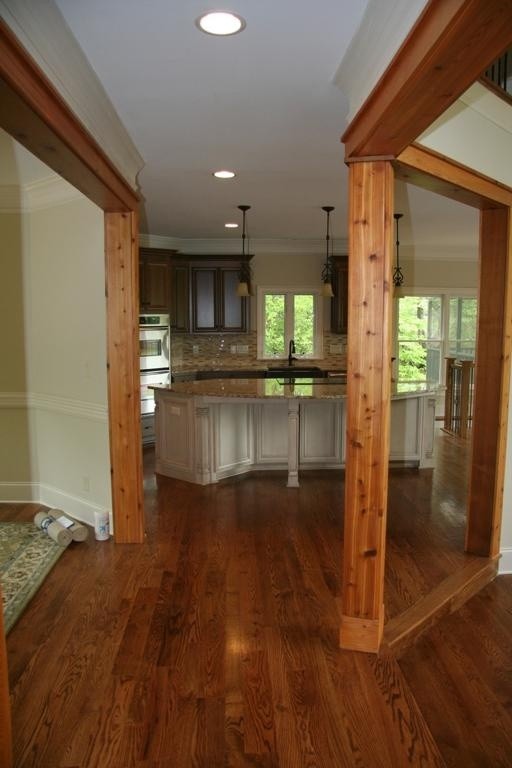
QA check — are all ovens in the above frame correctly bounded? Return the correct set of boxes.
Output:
[138,314,172,444]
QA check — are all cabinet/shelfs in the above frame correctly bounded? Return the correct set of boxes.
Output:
[141,413,156,449]
[170,253,191,336]
[139,247,177,315]
[328,255,348,333]
[192,254,255,336]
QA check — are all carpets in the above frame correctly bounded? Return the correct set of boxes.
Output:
[0,521,68,639]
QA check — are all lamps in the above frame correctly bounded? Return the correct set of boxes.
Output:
[237,205,251,297]
[392,213,405,298]
[319,206,335,296]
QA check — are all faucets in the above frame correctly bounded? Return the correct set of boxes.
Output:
[289,340,295,356]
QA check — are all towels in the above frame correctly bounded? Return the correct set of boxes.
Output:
[48,509,89,541]
[35,512,72,546]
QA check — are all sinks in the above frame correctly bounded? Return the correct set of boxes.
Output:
[265,368,321,378]
